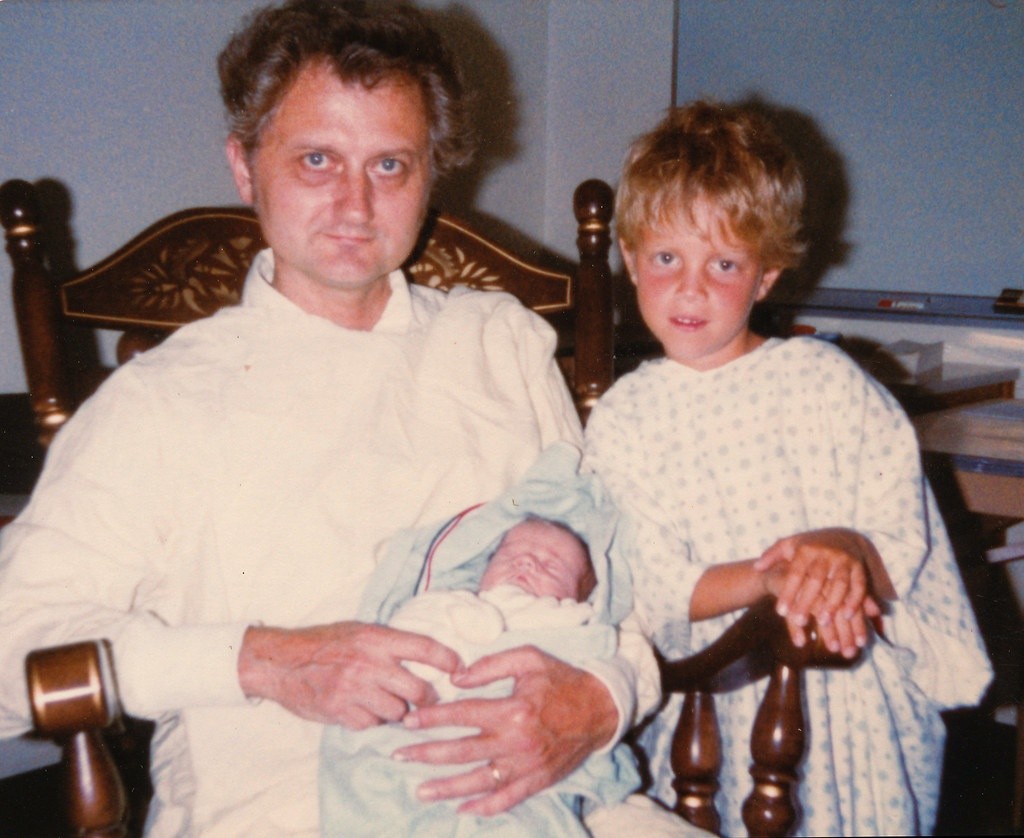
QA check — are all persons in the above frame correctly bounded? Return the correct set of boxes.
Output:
[312,441,619,836]
[0,1,665,835]
[578,91,999,838]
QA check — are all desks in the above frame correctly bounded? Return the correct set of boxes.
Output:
[910,397,1024,838]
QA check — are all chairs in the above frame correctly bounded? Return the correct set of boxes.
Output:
[0,176,861,837]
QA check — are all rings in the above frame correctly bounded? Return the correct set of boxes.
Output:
[491,766,502,783]
